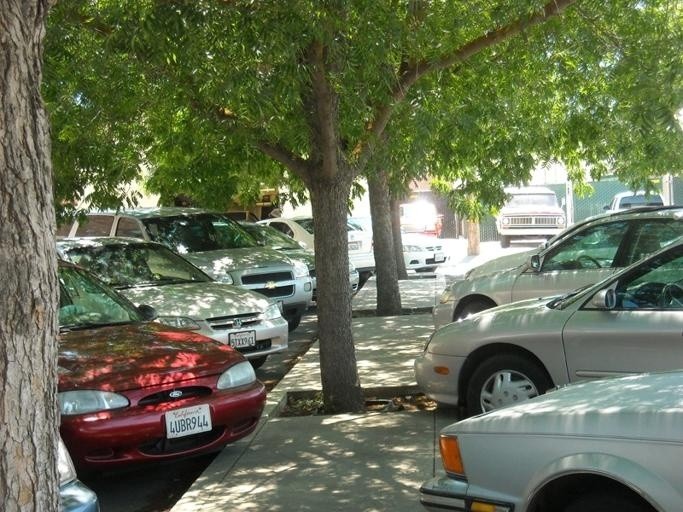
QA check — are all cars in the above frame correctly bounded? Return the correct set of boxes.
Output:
[419,369,683,512]
[431,206,683,331]
[414,236,683,420]
[603,191,664,213]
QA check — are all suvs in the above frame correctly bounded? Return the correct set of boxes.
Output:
[495,187,566,248]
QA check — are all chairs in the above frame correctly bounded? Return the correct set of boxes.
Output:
[632,234,660,263]
